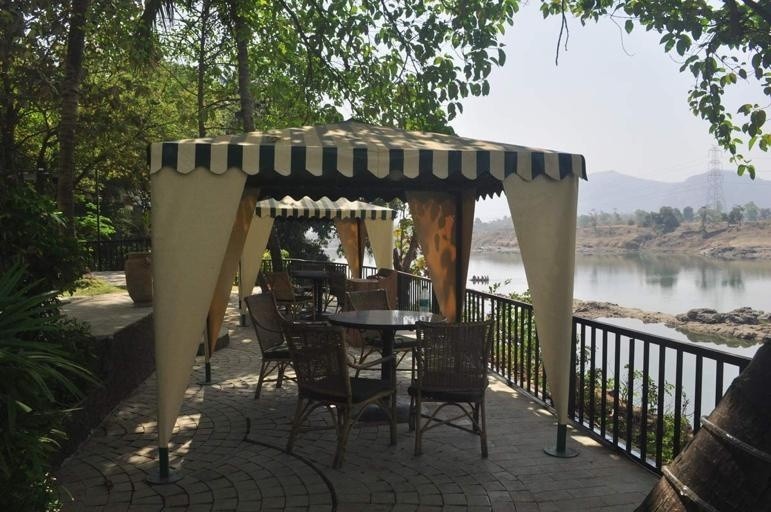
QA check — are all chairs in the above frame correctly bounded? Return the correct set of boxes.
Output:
[283,322,397,466]
[407,319,493,461]
[346,290,424,378]
[259,261,410,324]
[246,293,295,399]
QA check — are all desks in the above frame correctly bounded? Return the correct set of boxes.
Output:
[326,310,445,425]
